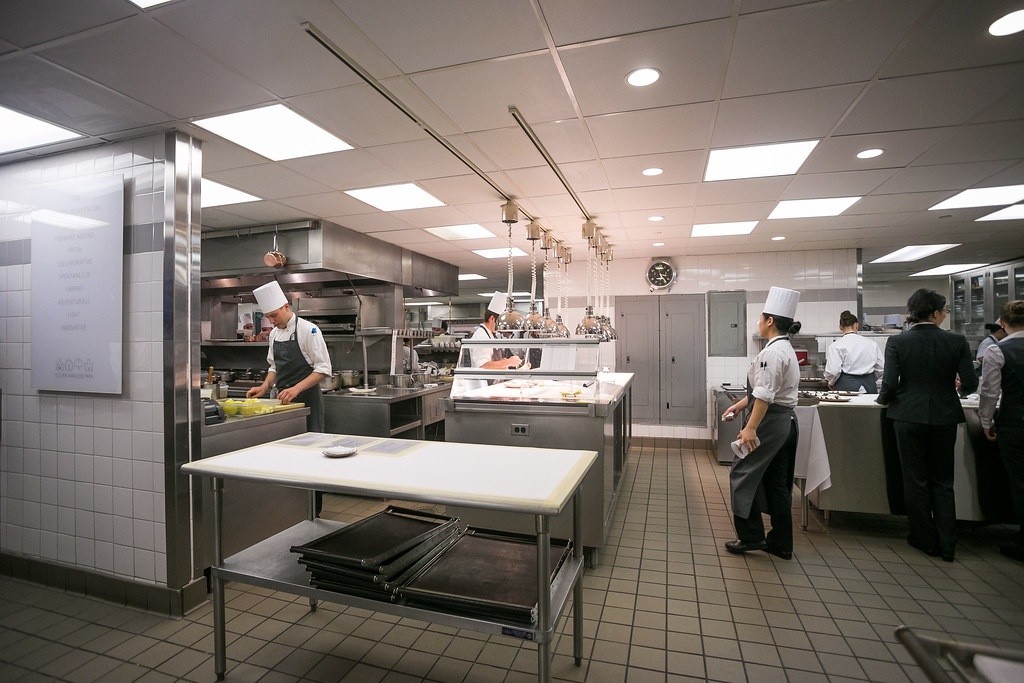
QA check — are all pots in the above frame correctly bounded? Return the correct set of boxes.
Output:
[263,235,287,266]
[318,369,360,393]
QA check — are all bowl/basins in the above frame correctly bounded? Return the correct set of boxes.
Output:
[215,397,281,418]
[393,374,413,388]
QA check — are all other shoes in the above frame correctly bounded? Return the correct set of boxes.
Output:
[1000,546,1024,561]
[762,543,792,560]
[907,534,931,553]
[926,544,955,562]
[725,540,768,551]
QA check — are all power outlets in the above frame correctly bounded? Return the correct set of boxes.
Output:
[510,424,529,436]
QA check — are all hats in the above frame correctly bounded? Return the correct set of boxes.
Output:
[253,281,288,314]
[995,318,1003,328]
[763,286,800,321]
[432,318,442,328]
[488,291,508,315]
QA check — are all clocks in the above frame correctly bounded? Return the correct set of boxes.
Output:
[645,259,677,291]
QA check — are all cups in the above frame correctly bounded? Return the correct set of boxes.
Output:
[730,436,761,459]
[244,329,254,337]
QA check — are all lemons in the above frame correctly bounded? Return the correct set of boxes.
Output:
[219,398,264,416]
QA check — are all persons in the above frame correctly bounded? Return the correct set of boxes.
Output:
[876,287,979,562]
[469,290,542,387]
[721,287,801,560]
[977,299,1024,566]
[975,316,1008,366]
[246,280,333,433]
[403,338,421,374]
[432,318,450,336]
[823,310,885,395]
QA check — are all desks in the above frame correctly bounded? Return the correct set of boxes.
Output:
[793,390,1001,531]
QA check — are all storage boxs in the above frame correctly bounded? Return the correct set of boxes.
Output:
[794,347,808,366]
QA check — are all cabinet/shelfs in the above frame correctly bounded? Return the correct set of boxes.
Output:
[323,389,452,441]
[948,257,1024,350]
[176,432,599,683]
[708,292,748,357]
[444,386,633,568]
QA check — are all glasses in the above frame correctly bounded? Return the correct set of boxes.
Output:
[432,328,436,330]
[942,308,951,313]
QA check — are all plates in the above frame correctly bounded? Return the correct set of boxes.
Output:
[322,447,357,457]
[797,390,862,402]
